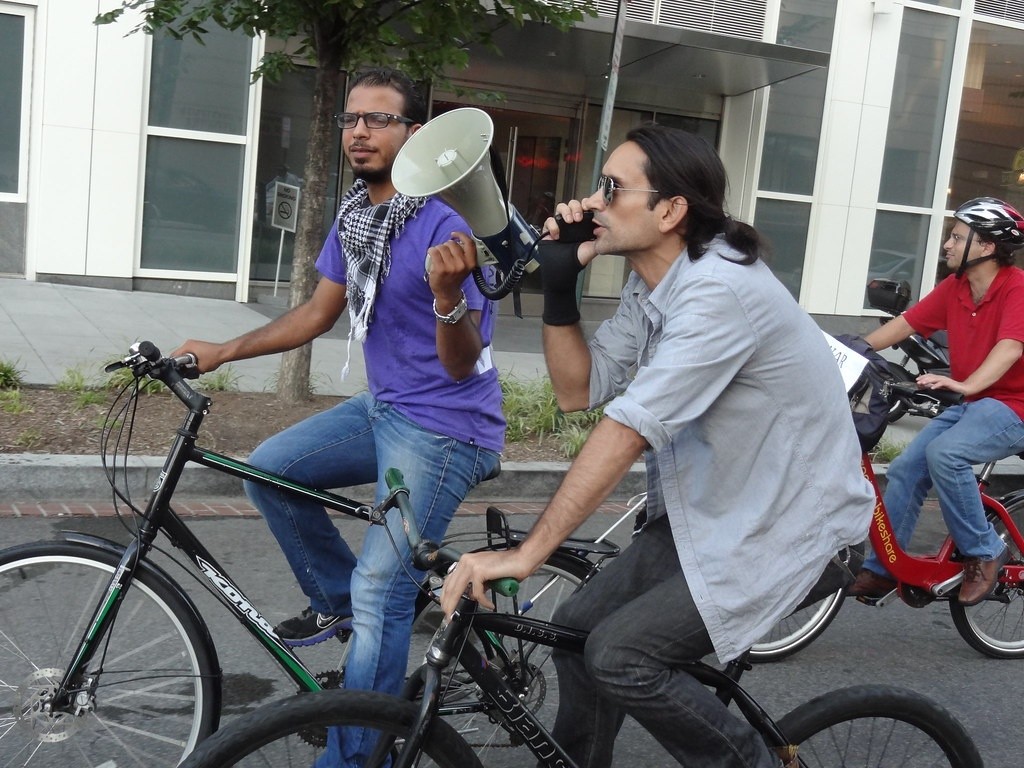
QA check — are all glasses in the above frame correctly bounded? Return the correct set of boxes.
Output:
[596,175,661,206]
[948,233,985,244]
[334,110,413,130]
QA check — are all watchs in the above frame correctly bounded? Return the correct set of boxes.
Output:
[432,288,468,326]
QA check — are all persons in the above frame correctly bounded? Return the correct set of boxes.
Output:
[844,195,1024,607]
[441,123,877,768]
[168,69,505,768]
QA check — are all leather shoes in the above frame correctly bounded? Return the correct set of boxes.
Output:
[845,568,893,596]
[959,545,1011,606]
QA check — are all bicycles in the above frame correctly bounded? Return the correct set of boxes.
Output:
[172,466,986,767]
[631,332,1024,663]
[0,335,624,768]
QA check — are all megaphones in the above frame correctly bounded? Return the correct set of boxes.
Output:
[391,107,544,278]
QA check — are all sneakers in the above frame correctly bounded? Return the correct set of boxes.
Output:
[274,607,355,648]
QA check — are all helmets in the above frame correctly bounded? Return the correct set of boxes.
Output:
[953,197,1024,249]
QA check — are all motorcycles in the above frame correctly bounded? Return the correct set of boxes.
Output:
[865,274,952,423]
